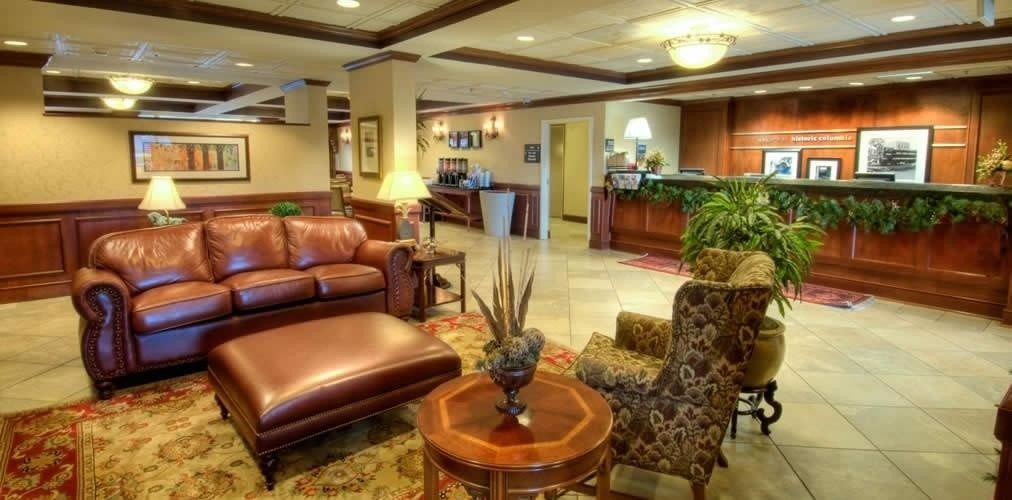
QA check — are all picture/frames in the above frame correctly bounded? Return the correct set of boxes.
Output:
[128,129,251,184]
[853,124,934,183]
[761,149,803,179]
[805,156,843,181]
[357,115,383,179]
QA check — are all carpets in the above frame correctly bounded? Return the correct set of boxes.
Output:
[619,251,873,311]
[1,308,577,500]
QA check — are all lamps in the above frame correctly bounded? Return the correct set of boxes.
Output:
[376,169,433,245]
[100,97,137,111]
[657,33,736,69]
[623,116,653,169]
[108,78,153,97]
[339,125,351,144]
[137,176,187,225]
[482,112,502,140]
[433,117,447,141]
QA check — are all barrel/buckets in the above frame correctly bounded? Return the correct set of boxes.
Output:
[481,190,515,236]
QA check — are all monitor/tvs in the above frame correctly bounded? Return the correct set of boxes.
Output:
[855,171,898,182]
[680,167,705,174]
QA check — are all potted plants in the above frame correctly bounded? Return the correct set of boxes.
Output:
[680,169,825,439]
[645,145,667,175]
[468,188,545,419]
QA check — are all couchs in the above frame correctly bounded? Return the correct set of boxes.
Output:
[208,311,463,491]
[564,248,775,500]
[71,213,416,400]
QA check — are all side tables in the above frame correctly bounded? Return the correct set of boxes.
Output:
[416,370,614,499]
[413,245,467,322]
